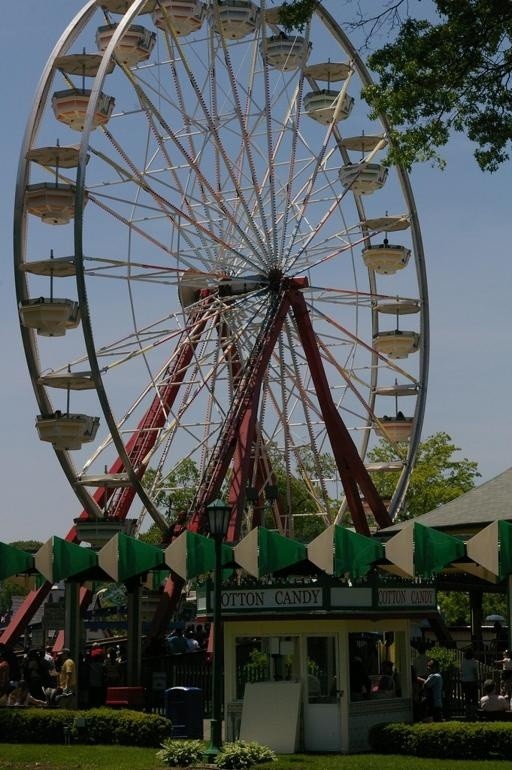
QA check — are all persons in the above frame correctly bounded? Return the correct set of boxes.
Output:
[350,620,511,720]
[1,646,76,705]
[77,622,213,711]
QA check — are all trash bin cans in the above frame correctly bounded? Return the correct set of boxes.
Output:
[164,685,203,739]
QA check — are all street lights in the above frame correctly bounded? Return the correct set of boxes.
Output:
[201,494,230,763]
